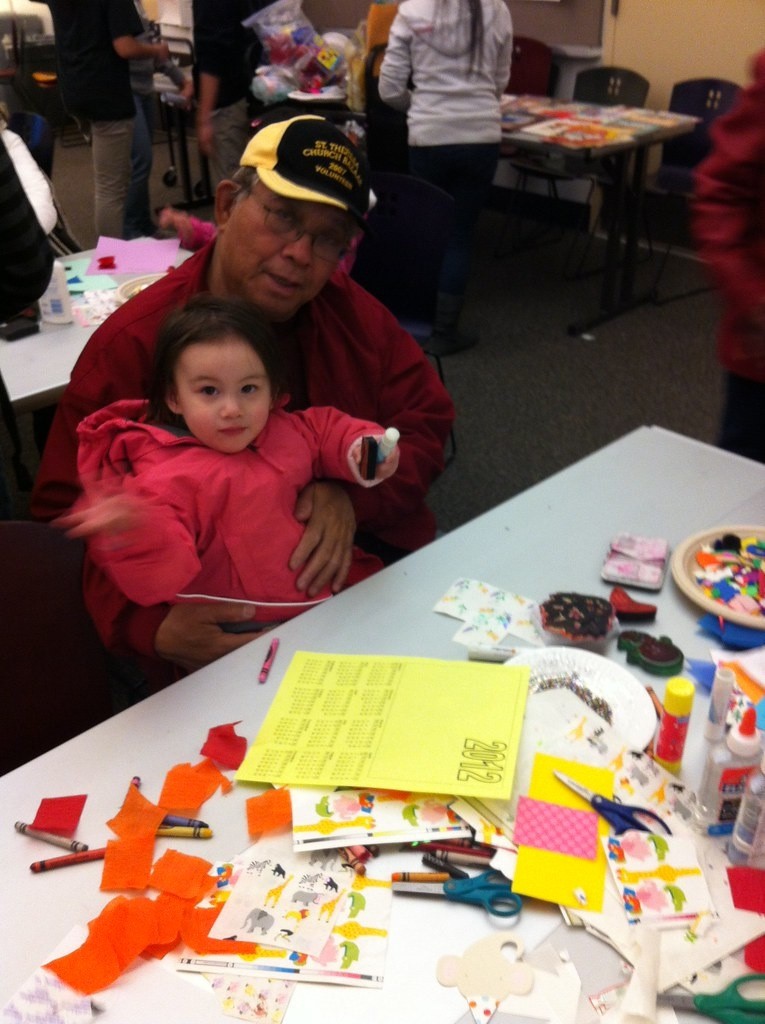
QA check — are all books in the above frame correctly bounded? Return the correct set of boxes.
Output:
[497,92,700,155]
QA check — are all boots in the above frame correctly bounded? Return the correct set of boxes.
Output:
[425,291,478,355]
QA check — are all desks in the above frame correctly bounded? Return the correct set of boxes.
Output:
[501,94,701,338]
[0,426,764,1023]
[0,236,195,414]
[153,65,214,216]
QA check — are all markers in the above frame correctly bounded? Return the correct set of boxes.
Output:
[470,644,528,662]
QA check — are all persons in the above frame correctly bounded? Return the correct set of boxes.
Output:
[26,114,454,704]
[47,290,400,636]
[692,45,765,466]
[0,0,513,355]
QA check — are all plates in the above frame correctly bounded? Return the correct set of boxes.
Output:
[116,273,168,304]
[671,524,765,632]
[288,92,347,102]
[504,647,657,750]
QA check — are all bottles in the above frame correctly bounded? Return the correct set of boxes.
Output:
[654,677,695,776]
[703,667,734,741]
[725,754,765,870]
[689,708,765,838]
[377,428,399,463]
[38,256,71,324]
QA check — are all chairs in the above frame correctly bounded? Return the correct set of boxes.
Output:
[351,36,745,468]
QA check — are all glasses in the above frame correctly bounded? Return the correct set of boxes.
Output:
[234,185,358,267]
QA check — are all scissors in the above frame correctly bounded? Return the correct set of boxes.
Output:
[393,868,522,916]
[555,769,671,836]
[657,973,765,1024]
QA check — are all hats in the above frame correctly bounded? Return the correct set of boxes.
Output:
[240,114,383,229]
